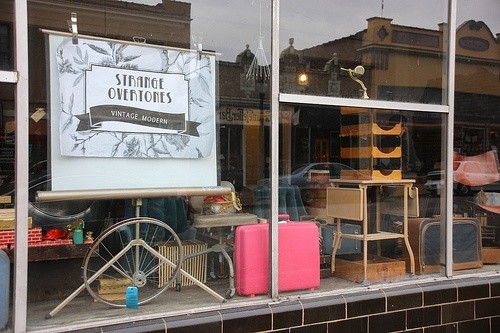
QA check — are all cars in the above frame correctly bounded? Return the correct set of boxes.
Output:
[255,162,371,195]
[422,160,500,197]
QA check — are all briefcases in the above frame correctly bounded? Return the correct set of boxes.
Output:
[232,214,321,299]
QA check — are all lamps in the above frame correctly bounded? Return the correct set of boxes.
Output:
[296,69,310,87]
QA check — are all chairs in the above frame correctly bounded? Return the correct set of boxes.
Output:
[172,182,259,301]
[113,197,197,280]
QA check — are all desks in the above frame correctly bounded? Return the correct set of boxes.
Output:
[326,179,421,287]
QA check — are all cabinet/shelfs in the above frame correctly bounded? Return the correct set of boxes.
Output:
[339,105,405,180]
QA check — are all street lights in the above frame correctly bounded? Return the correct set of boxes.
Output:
[254,76,269,181]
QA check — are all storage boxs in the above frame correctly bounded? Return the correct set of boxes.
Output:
[324,222,362,255]
[93,273,133,302]
[321,219,363,256]
[333,255,408,285]
